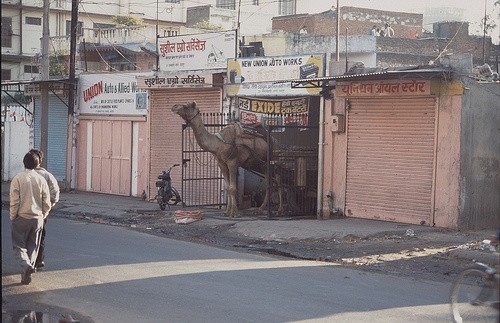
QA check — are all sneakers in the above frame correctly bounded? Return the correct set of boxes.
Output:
[35,261,45,271]
[21,266,35,284]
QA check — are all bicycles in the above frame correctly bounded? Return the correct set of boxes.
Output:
[155,163,181,211]
[448,240,500,323]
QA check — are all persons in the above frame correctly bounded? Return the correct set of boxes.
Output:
[29,149,60,268]
[9,152,51,284]
[300,26,307,33]
[371,23,394,38]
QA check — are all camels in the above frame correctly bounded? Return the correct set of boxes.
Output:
[171,101,284,219]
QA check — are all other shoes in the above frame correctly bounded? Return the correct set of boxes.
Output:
[492,302,500,309]
